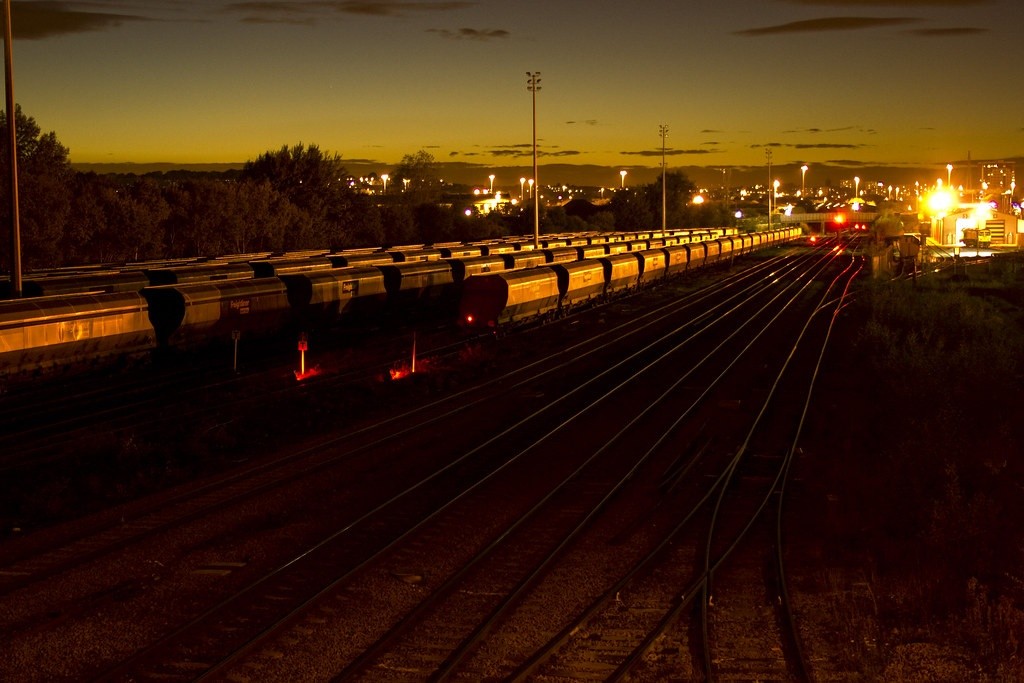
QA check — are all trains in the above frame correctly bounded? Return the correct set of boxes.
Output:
[0,222,803,399]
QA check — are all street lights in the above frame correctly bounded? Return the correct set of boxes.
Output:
[765,148,773,232]
[801,165,808,197]
[525,70,542,251]
[657,124,669,237]
[854,177,860,197]
